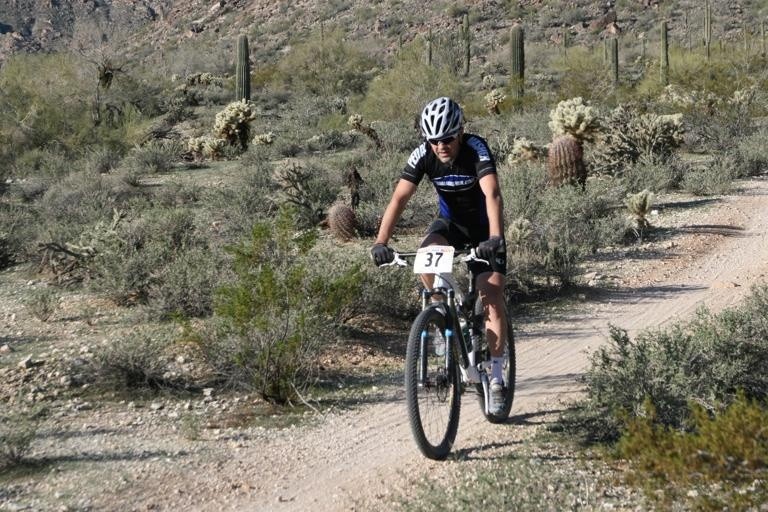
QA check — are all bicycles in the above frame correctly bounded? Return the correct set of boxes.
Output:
[366,247,516,459]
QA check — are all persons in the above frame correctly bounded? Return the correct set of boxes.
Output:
[369,97,510,416]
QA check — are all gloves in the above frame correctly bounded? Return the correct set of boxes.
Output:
[478,236,501,257]
[371,242,394,267]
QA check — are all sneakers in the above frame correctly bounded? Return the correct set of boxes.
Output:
[488,379,506,416]
[435,329,445,356]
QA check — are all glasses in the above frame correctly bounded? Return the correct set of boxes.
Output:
[429,137,455,145]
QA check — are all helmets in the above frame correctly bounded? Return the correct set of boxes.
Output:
[420,97,462,141]
